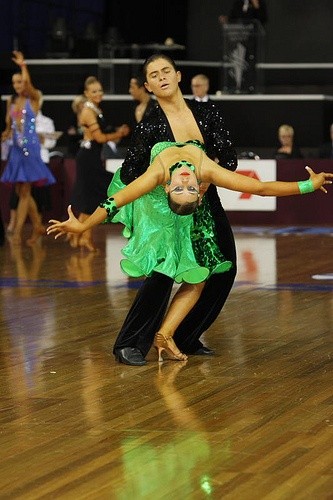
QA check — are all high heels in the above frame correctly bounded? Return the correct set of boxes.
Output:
[78,237,99,252]
[64,231,77,248]
[152,332,188,362]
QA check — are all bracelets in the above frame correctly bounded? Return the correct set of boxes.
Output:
[297,180,314,194]
[20,64,26,66]
[100,197,117,216]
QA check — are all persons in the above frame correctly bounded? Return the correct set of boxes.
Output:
[190,74,221,108]
[118,77,159,148]
[6,244,46,384]
[112,54,238,366]
[69,76,129,253]
[2,50,56,243]
[114,360,214,500]
[46,140,333,361]
[273,122,333,159]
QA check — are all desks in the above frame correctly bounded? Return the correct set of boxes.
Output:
[0,157,333,226]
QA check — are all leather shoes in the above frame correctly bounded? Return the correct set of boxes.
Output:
[113,347,146,366]
[174,337,215,355]
